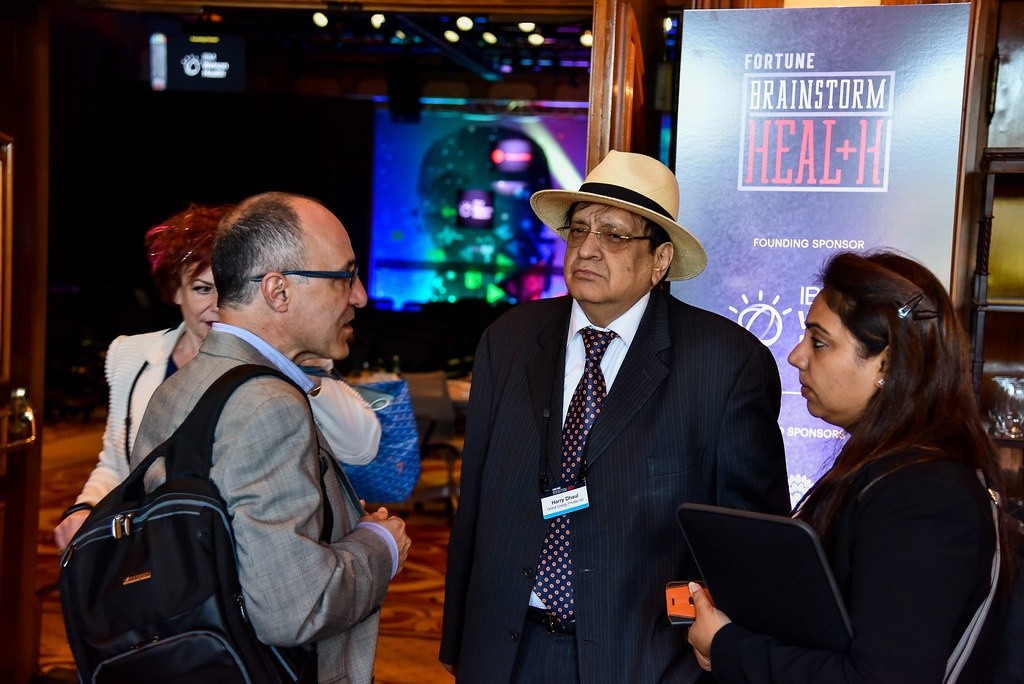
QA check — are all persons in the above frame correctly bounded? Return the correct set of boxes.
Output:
[129,191,411,684]
[53,205,382,558]
[437,150,792,684]
[686,247,1016,684]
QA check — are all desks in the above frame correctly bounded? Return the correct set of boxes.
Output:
[406,401,472,509]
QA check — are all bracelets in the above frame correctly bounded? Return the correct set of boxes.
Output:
[308,385,322,394]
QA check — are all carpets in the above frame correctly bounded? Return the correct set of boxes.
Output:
[40,457,451,639]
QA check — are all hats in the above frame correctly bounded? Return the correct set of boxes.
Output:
[529,150,707,281]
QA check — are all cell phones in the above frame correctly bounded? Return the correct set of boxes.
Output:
[667,580,707,624]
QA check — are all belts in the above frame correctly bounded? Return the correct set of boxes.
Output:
[527,614,575,634]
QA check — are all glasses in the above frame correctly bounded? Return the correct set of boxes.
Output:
[556,225,650,253]
[251,266,360,290]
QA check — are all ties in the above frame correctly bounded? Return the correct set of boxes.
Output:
[532,326,621,624]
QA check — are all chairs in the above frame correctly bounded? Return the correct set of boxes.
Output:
[397,373,461,526]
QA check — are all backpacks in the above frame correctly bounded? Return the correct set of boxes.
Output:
[57,364,333,684]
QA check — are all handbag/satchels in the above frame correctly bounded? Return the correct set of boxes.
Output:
[300,358,420,502]
